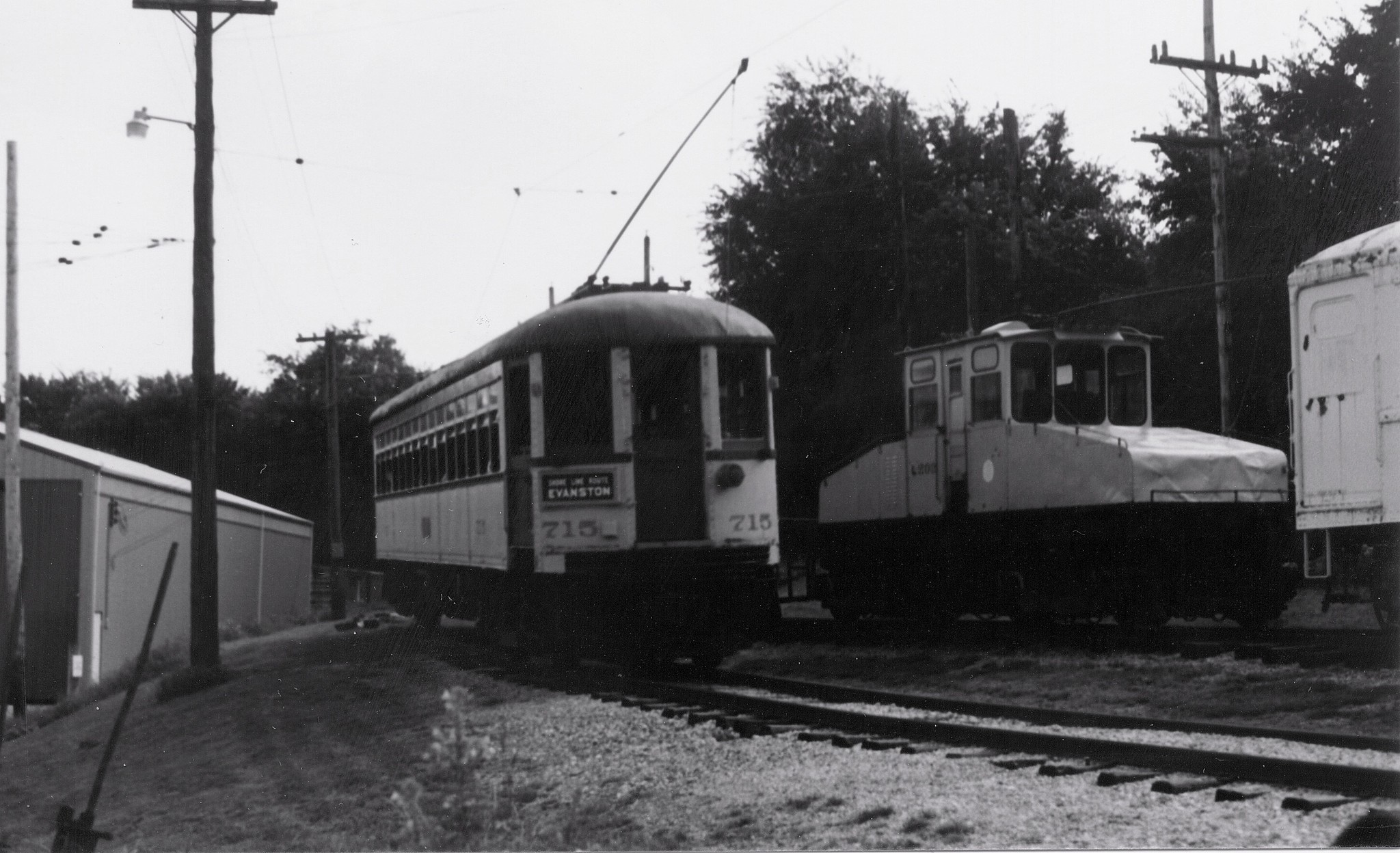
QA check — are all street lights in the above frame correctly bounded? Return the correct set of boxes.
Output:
[122,109,226,675]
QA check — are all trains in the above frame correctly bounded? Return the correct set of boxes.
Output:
[811,217,1400,614]
[369,235,784,649]
